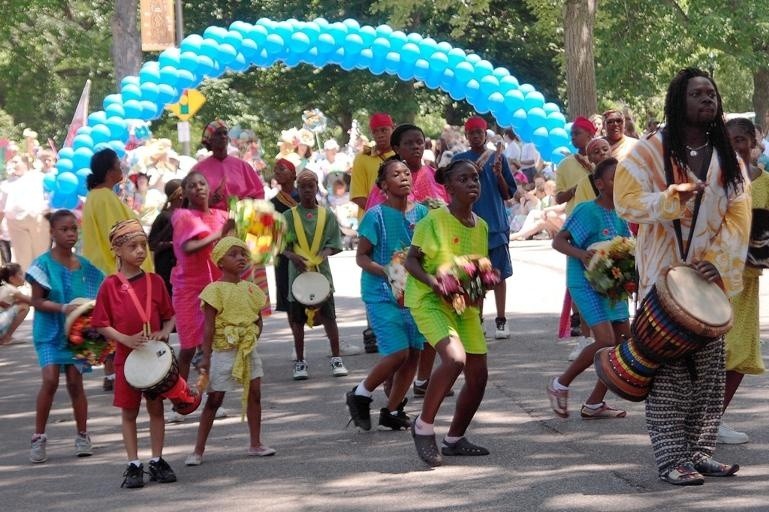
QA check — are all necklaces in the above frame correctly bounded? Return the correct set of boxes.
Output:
[683,140,709,157]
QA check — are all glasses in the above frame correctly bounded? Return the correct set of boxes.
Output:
[606,118,623,126]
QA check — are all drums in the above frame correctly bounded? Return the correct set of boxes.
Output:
[123,339,202,415]
[593,261,733,402]
[292,271,331,325]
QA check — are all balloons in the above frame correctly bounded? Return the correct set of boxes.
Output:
[39,18,578,213]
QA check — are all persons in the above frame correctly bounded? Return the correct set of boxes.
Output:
[547,108,661,419]
[613,67,739,487]
[749,123,769,173]
[716,118,768,447]
[3,115,568,486]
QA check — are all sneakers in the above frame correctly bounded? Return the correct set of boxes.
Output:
[545,313,749,487]
[345,314,511,467]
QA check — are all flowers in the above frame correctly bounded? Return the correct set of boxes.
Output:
[62,309,114,369]
[435,253,502,316]
[226,197,288,267]
[584,233,640,303]
